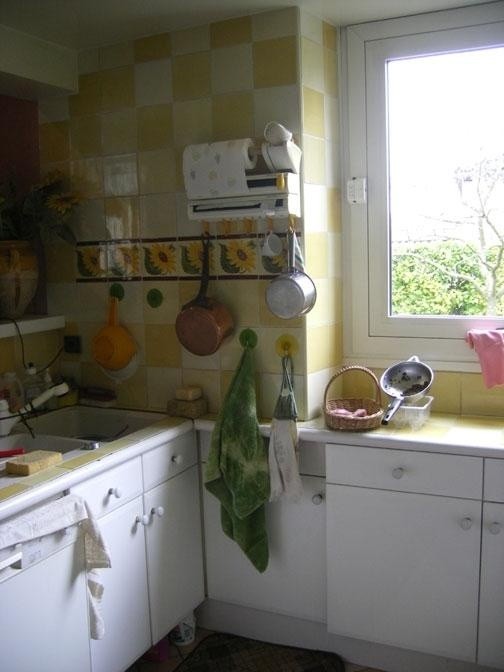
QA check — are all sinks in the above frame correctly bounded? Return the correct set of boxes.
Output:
[1,429,84,457]
[12,404,169,444]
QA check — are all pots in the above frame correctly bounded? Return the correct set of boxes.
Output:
[264,228,318,321]
[90,294,138,372]
[175,231,235,358]
[378,354,432,424]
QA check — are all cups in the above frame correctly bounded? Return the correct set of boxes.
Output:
[261,228,282,259]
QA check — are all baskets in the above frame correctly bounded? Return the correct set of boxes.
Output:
[323,365,385,433]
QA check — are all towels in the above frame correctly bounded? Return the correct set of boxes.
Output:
[203,339,305,574]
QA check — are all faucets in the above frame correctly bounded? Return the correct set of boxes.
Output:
[1,382,68,437]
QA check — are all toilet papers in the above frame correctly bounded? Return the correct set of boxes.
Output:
[182,139,257,202]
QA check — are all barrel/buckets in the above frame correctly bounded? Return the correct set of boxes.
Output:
[0,371,25,412]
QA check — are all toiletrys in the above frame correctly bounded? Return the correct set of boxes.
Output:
[21,361,44,411]
[42,367,57,409]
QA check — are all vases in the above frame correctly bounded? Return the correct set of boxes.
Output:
[0,239,39,320]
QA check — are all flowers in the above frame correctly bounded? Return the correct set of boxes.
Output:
[0,167,87,320]
